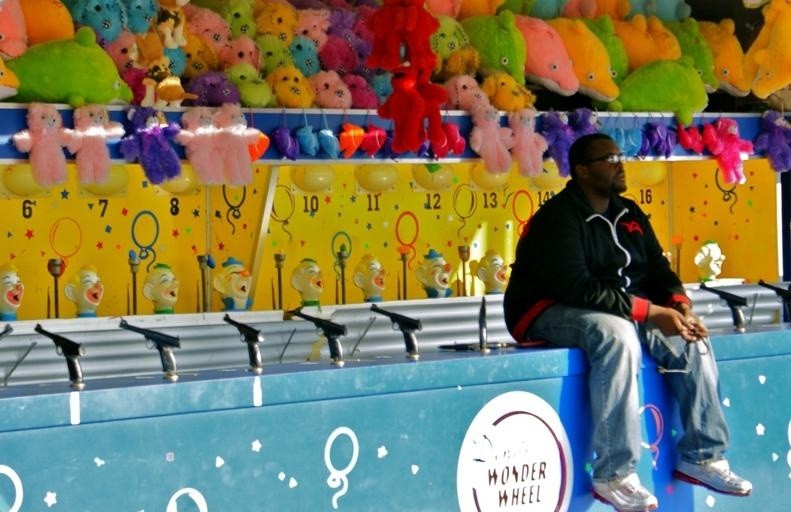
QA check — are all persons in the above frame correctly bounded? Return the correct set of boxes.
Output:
[501,132,755,511]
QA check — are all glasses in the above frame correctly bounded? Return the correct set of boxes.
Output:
[589,153,626,165]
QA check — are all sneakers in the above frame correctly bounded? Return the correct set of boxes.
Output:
[590,468,659,512]
[671,456,752,497]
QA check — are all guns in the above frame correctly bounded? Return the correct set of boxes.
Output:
[292,309,347,359]
[120,319,179,372]
[439,340,511,350]
[223,313,264,369]
[371,304,422,354]
[478,296,488,348]
[757,279,791,321]
[35,324,86,389]
[699,281,748,326]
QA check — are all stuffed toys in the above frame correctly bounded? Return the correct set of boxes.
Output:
[0,0,791,189]
[0,2,790,191]
